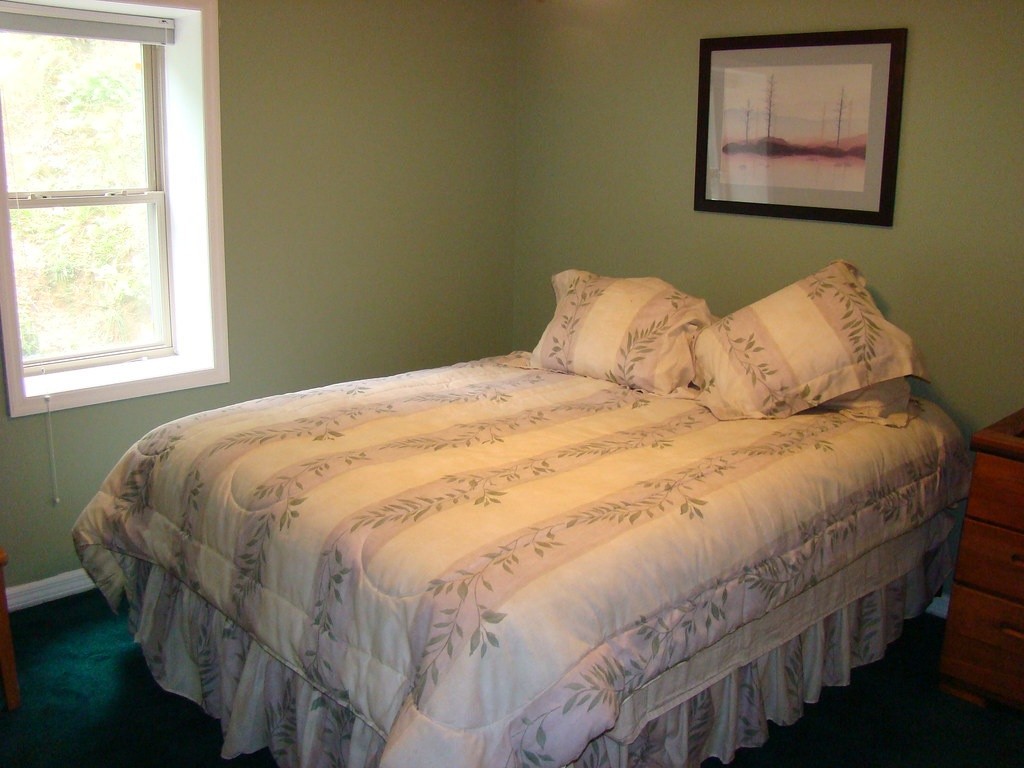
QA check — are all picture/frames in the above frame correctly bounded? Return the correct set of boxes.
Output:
[693,25,908,227]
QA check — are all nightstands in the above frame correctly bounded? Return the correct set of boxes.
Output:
[939,409,1024,720]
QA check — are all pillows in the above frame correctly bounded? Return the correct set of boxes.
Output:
[815,374,911,428]
[695,260,931,421]
[531,269,723,394]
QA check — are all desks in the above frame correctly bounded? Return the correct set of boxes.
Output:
[71,347,972,768]
